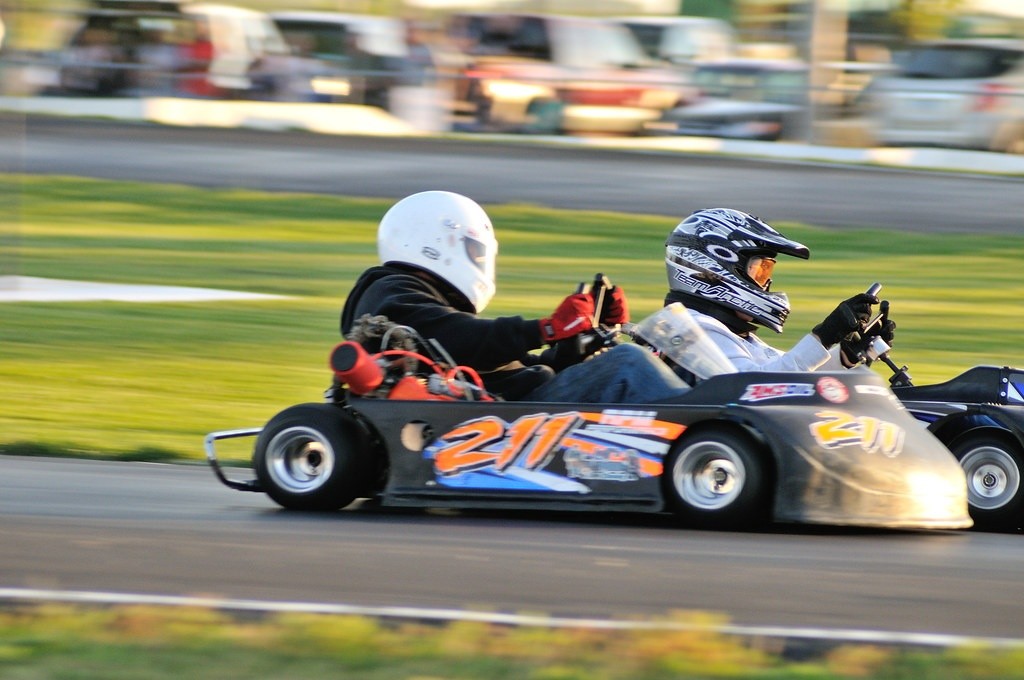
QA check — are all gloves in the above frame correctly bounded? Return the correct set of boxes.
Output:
[538,293,596,341]
[811,293,880,350]
[588,285,631,326]
[839,300,896,370]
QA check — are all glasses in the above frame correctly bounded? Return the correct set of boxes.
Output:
[746,256,775,287]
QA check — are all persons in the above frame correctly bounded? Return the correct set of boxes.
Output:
[321,188,694,407]
[630,205,898,388]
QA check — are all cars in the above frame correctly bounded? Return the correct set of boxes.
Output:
[52,0,314,100]
[416,8,809,149]
[852,40,1024,154]
[269,17,440,113]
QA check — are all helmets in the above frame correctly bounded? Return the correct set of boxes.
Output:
[376,190,499,314]
[664,208,810,335]
